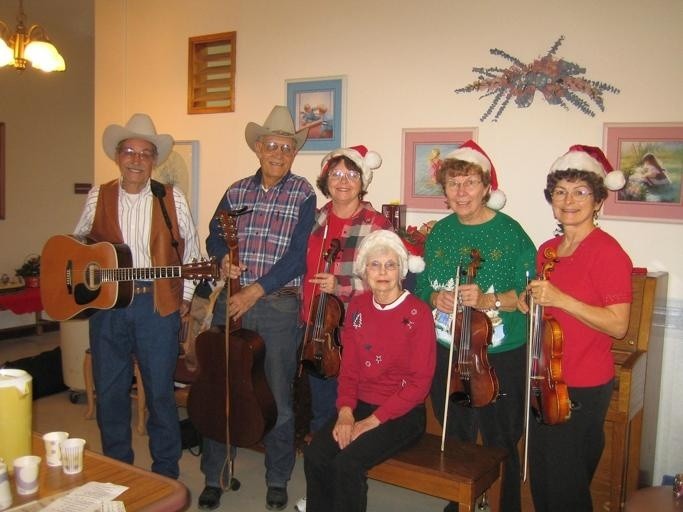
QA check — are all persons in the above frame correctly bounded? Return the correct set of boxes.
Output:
[294,146,396,512]
[72,114,202,480]
[305,232,436,512]
[414,140,537,512]
[518,145,632,512]
[198,106,316,511]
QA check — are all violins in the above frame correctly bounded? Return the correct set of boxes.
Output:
[296,239,345,379]
[446,249,499,408]
[531,248,569,426]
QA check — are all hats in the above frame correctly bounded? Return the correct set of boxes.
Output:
[245,105,309,152]
[548,144,626,191]
[444,139,506,210]
[352,230,425,280]
[320,144,382,192]
[103,113,174,168]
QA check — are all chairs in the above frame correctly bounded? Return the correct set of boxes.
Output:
[83,348,146,434]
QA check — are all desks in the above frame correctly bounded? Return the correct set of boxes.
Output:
[1,430,192,512]
[0,286,44,336]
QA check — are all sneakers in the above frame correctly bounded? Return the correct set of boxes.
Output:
[296,495,311,512]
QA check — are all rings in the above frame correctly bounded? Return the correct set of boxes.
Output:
[323,284,327,288]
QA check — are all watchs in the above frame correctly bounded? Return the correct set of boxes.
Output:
[494,293,501,311]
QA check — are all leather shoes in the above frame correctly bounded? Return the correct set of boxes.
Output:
[265,487,288,509]
[198,486,222,510]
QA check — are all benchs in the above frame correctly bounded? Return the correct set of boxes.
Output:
[175,384,512,512]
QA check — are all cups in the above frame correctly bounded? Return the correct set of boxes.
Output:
[13,455,41,495]
[42,431,69,466]
[59,438,86,474]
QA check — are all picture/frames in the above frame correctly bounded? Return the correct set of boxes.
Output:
[285,74,347,155]
[400,128,478,214]
[597,122,683,224]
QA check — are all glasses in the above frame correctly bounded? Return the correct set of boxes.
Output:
[450,179,481,189]
[327,169,361,181]
[550,185,593,200]
[119,147,153,160]
[257,140,294,155]
[364,259,400,271]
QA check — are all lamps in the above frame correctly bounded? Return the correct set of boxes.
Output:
[0,0,66,73]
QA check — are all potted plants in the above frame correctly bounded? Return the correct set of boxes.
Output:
[14,253,40,288]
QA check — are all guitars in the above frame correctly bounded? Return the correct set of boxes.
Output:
[186,210,278,448]
[39,234,220,321]
